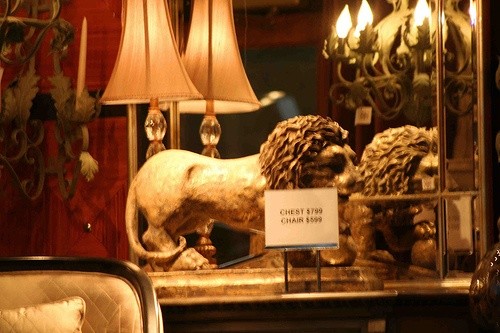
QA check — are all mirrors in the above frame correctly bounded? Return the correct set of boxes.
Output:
[122,0,486,280]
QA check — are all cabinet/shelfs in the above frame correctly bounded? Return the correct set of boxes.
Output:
[150,273,500,333]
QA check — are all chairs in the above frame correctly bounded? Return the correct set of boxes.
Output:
[0,255,165,333]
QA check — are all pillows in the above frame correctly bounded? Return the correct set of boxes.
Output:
[1,295,87,332]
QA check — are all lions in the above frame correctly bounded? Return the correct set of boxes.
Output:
[125,115,365,271]
[342,125,440,277]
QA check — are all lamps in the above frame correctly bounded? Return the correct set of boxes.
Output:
[100,1,206,265]
[322,1,479,129]
[173,0,263,268]
